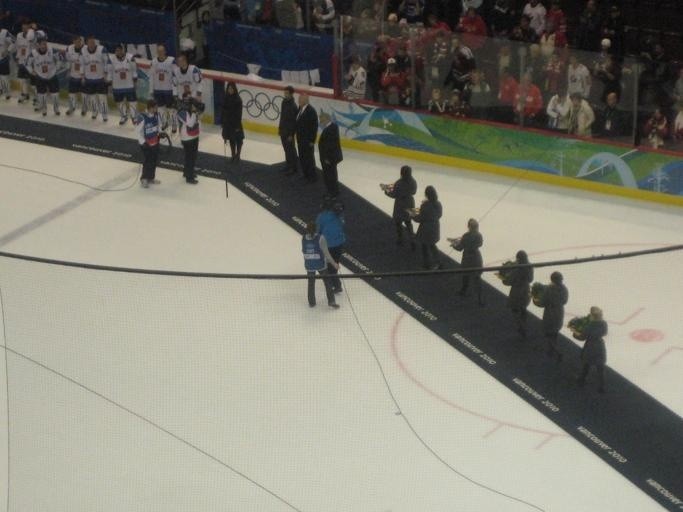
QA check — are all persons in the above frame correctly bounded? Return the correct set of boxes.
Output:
[410,186,442,268]
[221,0,683,194]
[315,195,345,294]
[302,217,340,308]
[502,251,533,337]
[534,272,569,354]
[452,219,482,297]
[572,307,607,383]
[0,24,205,188]
[385,165,417,247]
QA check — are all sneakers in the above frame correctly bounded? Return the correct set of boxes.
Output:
[119,116,128,125]
[149,180,160,185]
[183,172,195,178]
[329,303,339,308]
[334,288,342,293]
[142,179,148,188]
[188,179,198,184]
[132,117,142,125]
[0,89,48,116]
[54,107,106,121]
